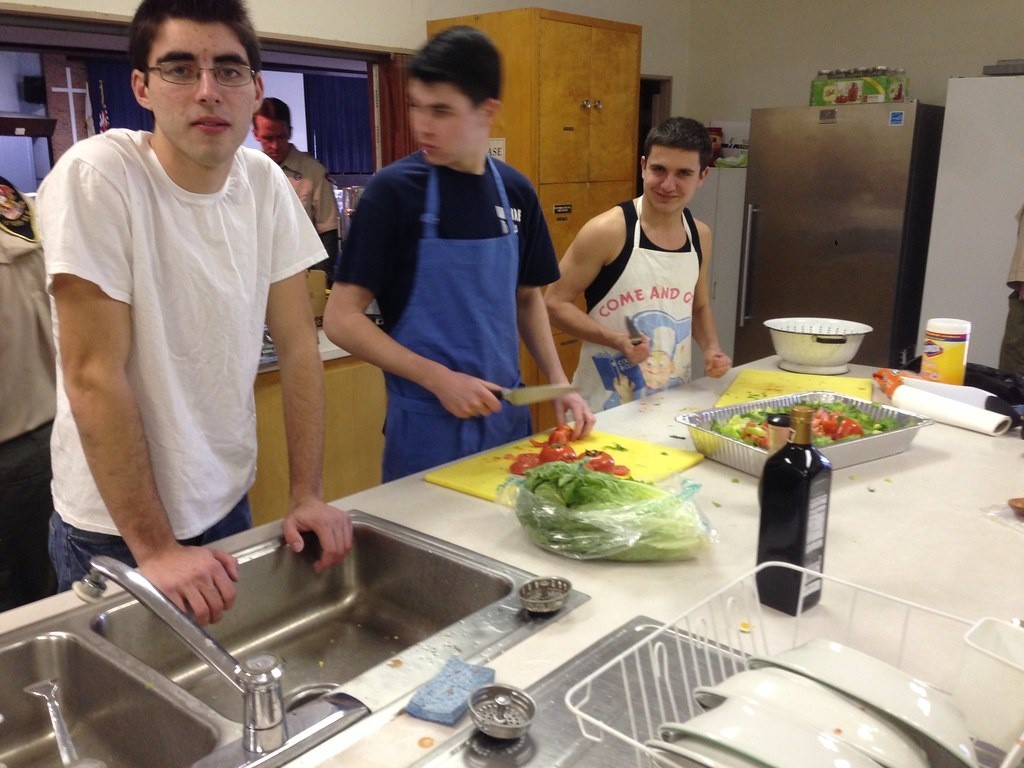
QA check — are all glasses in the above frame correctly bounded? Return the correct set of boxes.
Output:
[145,61,257,87]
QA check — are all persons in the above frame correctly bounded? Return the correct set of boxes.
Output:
[0,176,57,614]
[543,116,732,425]
[251,97,341,286]
[322,25,596,484]
[35,0,353,624]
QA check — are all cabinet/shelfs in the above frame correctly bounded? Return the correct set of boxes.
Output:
[426,8,643,436]
[685,168,747,382]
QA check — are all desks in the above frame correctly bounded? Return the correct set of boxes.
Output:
[249,325,388,529]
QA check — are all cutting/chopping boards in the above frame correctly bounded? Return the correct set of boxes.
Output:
[423,423,706,510]
[713,367,874,420]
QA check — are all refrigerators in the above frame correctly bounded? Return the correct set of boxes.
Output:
[729,99,949,373]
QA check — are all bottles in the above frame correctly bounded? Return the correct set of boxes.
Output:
[810,65,908,104]
[756,406,833,615]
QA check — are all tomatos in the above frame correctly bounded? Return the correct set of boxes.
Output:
[510,424,629,475]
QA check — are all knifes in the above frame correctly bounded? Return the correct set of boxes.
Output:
[624,315,646,348]
[486,383,585,408]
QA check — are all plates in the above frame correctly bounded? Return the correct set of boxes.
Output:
[638,638,977,768]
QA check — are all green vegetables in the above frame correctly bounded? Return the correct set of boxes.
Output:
[516,461,706,561]
[709,398,898,448]
